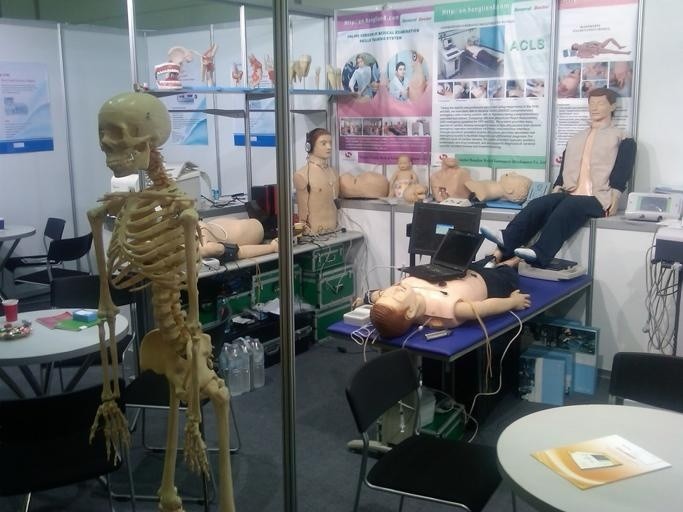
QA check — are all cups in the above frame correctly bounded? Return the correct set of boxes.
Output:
[2,299,18,321]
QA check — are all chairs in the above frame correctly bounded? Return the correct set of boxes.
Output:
[12,230,93,289]
[0,378,135,512]
[37,273,138,398]
[343,348,518,510]
[107,320,244,512]
[3,216,68,273]
[605,350,683,414]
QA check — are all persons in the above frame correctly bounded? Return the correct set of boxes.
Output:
[387,155,418,199]
[409,49,426,100]
[350,247,532,338]
[479,87,637,271]
[463,43,501,64]
[372,59,380,83]
[436,78,546,98]
[429,154,472,206]
[462,171,532,205]
[293,127,339,236]
[347,54,372,99]
[570,37,631,58]
[340,119,406,138]
[388,61,408,102]
[190,212,299,264]
[557,61,632,98]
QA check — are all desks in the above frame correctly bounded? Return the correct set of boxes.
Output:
[0,306,133,512]
[0,225,36,308]
[323,249,595,465]
[133,226,364,340]
[495,403,682,512]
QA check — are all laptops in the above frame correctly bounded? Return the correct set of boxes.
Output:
[397,228,485,282]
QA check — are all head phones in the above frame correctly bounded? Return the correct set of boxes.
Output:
[304,131,313,154]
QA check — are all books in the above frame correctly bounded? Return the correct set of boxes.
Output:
[529,432,673,492]
[53,309,106,333]
[36,311,73,331]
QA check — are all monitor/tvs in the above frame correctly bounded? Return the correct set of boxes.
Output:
[407,202,481,261]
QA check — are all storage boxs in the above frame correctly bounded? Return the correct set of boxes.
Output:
[299,297,353,341]
[249,263,304,306]
[181,290,255,331]
[300,263,356,308]
[294,245,345,272]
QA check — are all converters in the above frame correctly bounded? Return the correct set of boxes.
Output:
[333,199,341,210]
